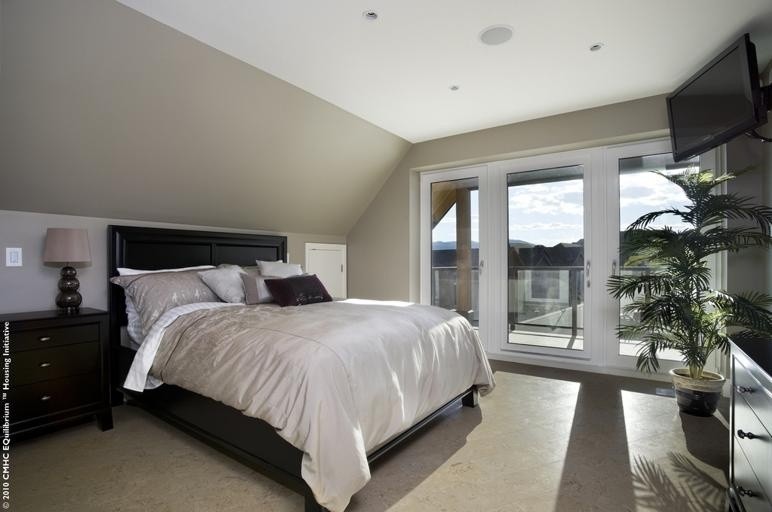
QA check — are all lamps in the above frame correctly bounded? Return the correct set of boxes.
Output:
[43,228,92,315]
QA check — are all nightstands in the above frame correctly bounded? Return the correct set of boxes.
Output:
[0,307,114,443]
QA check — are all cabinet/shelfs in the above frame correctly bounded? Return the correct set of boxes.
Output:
[727,334,772,512]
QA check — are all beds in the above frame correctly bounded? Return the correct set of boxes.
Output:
[106,224,494,512]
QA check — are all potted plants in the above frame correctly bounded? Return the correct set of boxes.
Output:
[605,167,772,418]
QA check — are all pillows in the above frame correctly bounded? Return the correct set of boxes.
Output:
[110,260,333,343]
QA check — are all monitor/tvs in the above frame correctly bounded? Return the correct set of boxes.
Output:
[665,33,768,163]
